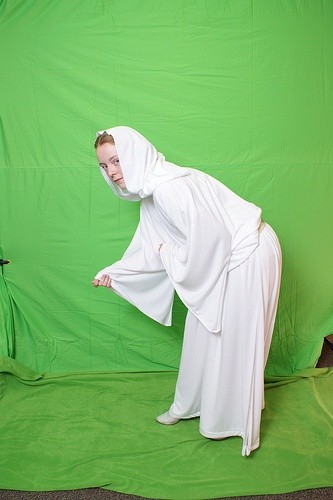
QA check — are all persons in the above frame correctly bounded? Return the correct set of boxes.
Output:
[90,125,283,456]
